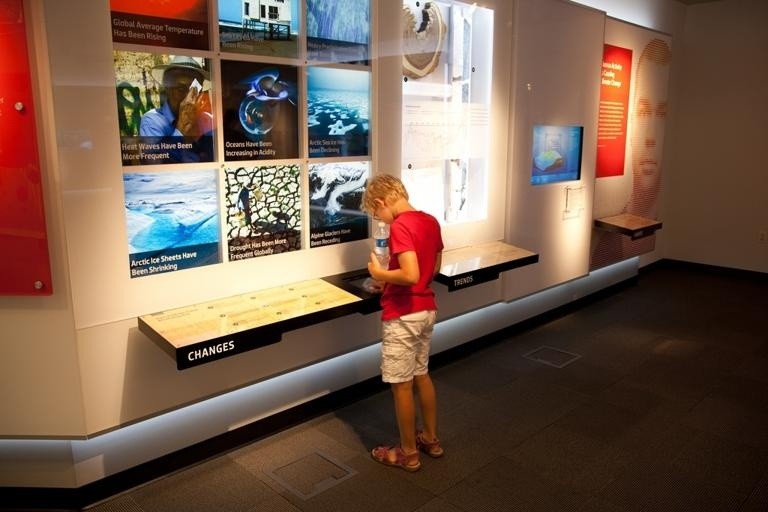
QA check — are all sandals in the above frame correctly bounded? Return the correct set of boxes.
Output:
[372,443,421,472]
[416,431,444,458]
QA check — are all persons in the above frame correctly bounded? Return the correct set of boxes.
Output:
[139,56,214,165]
[360,172,445,472]
[592,39,673,268]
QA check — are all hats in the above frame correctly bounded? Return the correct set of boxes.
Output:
[151,55,210,92]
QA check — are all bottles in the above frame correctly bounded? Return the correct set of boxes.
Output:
[374,221,390,270]
